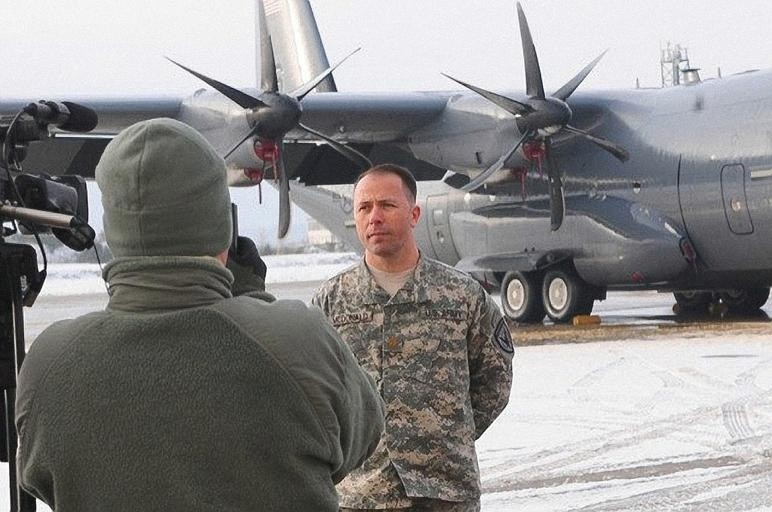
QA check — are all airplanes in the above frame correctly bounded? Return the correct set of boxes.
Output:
[0,0,772,328]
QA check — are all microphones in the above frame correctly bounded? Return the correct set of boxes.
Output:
[27,99,98,133]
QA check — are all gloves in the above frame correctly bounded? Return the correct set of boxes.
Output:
[228,237,267,280]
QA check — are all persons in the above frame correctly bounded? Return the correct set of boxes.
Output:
[13,114,390,511]
[303,162,518,511]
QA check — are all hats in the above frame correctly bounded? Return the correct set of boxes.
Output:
[95,117,234,256]
